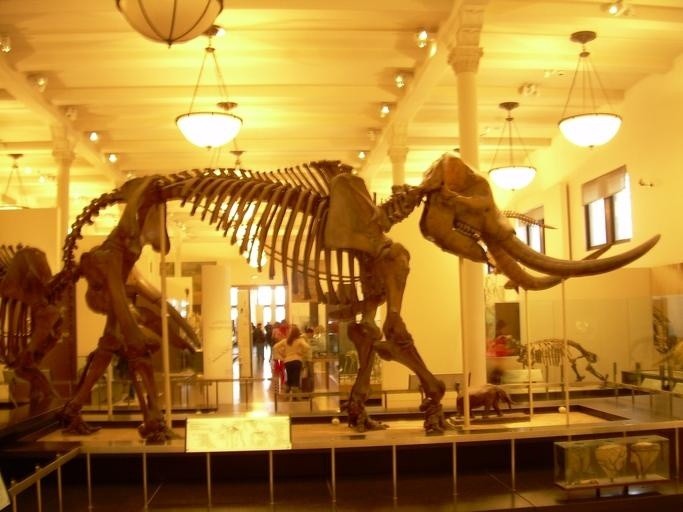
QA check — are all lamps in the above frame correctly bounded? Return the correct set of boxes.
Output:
[487,31,623,194]
[115,1,243,150]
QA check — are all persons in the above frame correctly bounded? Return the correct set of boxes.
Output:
[250,320,315,400]
